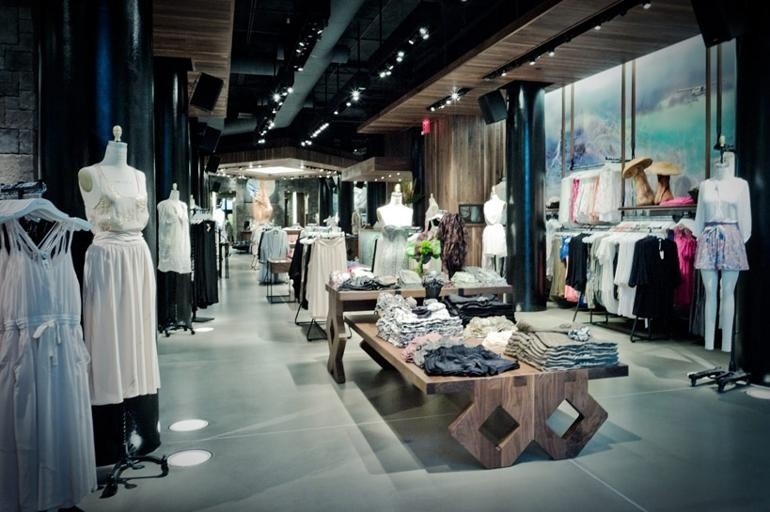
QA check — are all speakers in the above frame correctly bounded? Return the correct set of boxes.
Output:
[212,181,221,192]
[690,0,751,49]
[207,155,220,173]
[201,125,222,153]
[478,89,510,125]
[190,72,225,114]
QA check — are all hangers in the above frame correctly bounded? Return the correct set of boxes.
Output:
[0,178,94,233]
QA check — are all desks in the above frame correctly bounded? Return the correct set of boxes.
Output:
[341,310,630,470]
[326,280,512,386]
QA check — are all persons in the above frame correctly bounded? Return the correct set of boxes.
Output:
[376,191,413,287]
[491,176,510,221]
[694,163,752,353]
[77,138,162,463]
[482,191,508,277]
[158,189,190,275]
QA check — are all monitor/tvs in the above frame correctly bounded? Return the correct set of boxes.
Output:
[458,204,484,224]
